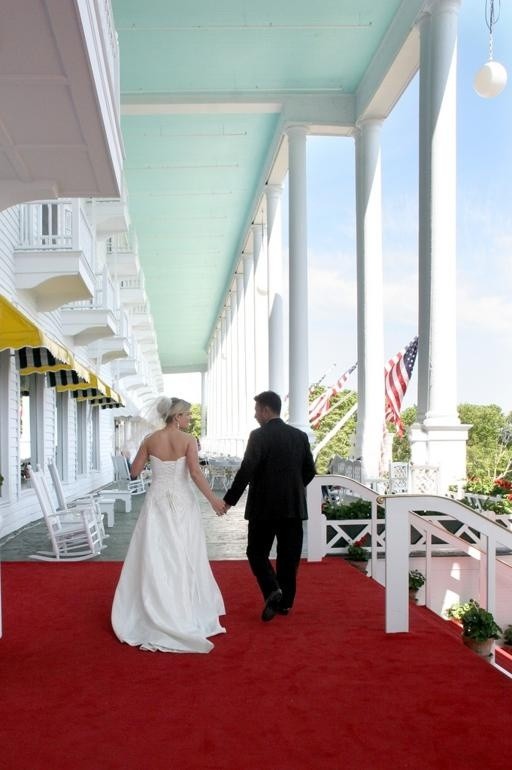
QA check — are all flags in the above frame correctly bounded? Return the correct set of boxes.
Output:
[310,356,358,428]
[384,335,420,444]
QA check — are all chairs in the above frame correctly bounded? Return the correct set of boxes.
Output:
[26,458,110,562]
[205,457,229,492]
[110,452,147,495]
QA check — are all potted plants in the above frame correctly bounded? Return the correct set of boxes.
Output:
[409,568,426,604]
[445,597,512,665]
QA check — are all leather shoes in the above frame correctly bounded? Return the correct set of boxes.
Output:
[260,589,292,621]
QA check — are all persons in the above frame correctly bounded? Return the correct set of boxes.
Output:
[221,391,316,622]
[111,395,228,656]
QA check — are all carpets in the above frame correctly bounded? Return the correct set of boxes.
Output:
[1,559,510,770]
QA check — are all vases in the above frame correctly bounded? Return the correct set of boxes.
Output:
[350,561,368,575]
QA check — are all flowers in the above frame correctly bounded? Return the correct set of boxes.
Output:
[347,536,369,561]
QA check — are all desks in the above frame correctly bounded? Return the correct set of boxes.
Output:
[72,489,134,527]
